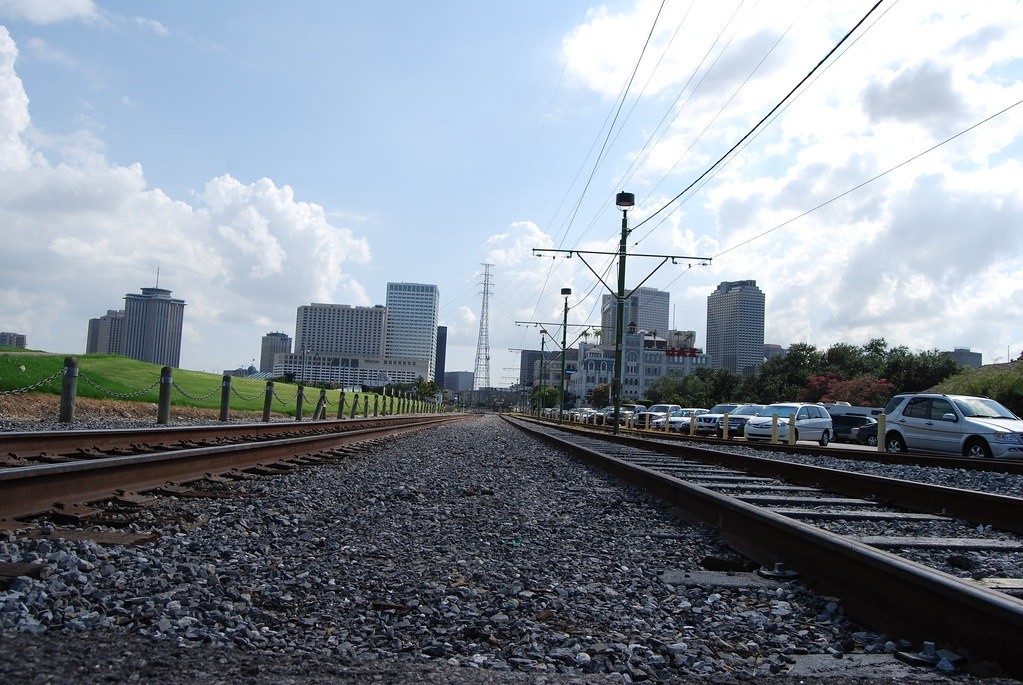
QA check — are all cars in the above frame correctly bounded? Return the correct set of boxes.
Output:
[856,422,877,446]
[884,393,1023,462]
[715,404,766,439]
[542,408,595,423]
[652,408,709,433]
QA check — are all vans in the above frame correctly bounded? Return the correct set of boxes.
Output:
[591,404,646,428]
[744,403,832,448]
[829,413,877,444]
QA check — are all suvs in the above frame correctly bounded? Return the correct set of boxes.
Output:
[696,403,743,436]
[637,404,682,430]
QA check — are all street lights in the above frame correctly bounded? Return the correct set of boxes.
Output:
[301,348,310,384]
[352,366,356,392]
[517,379,519,411]
[560,288,571,422]
[537,330,547,420]
[613,190,636,434]
[329,358,334,386]
[457,393,461,409]
[342,362,346,390]
[313,352,319,386]
